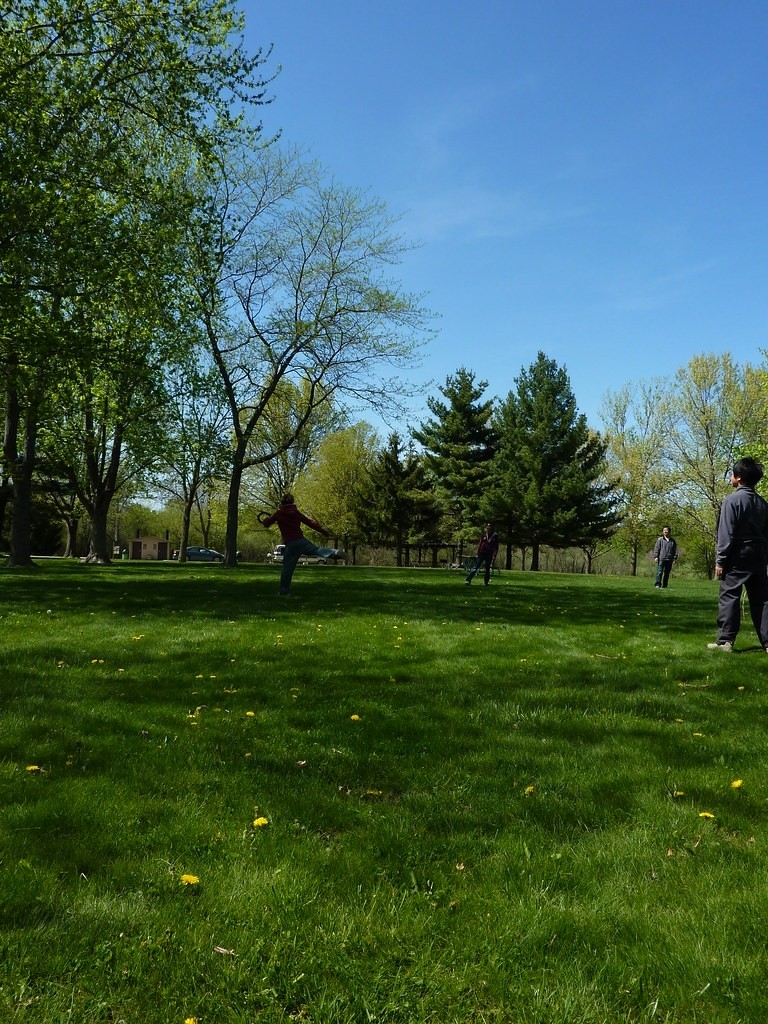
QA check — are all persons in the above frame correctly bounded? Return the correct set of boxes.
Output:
[707,457,768,655]
[652,526,678,588]
[81,525,116,565]
[465,524,499,587]
[263,493,350,599]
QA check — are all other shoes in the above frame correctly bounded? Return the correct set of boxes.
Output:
[275,592,291,598]
[484,583,489,587]
[334,550,349,561]
[707,642,733,652]
[465,580,470,585]
[655,586,659,589]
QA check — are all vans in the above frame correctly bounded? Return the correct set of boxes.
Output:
[272,544,327,566]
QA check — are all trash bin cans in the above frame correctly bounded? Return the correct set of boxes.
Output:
[122,549,129,560]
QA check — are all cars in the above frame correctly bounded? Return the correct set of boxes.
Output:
[173,545,226,563]
